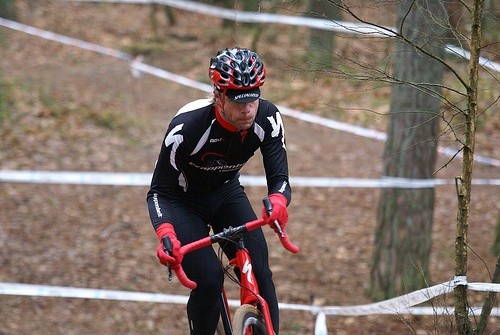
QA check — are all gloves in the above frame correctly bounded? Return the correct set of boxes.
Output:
[262,193,289,234]
[155,222,183,271]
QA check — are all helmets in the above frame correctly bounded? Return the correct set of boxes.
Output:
[207,47,266,104]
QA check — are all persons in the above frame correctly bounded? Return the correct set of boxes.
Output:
[146,48,293,335]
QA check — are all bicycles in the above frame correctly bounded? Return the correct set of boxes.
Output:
[163,197,300,335]
